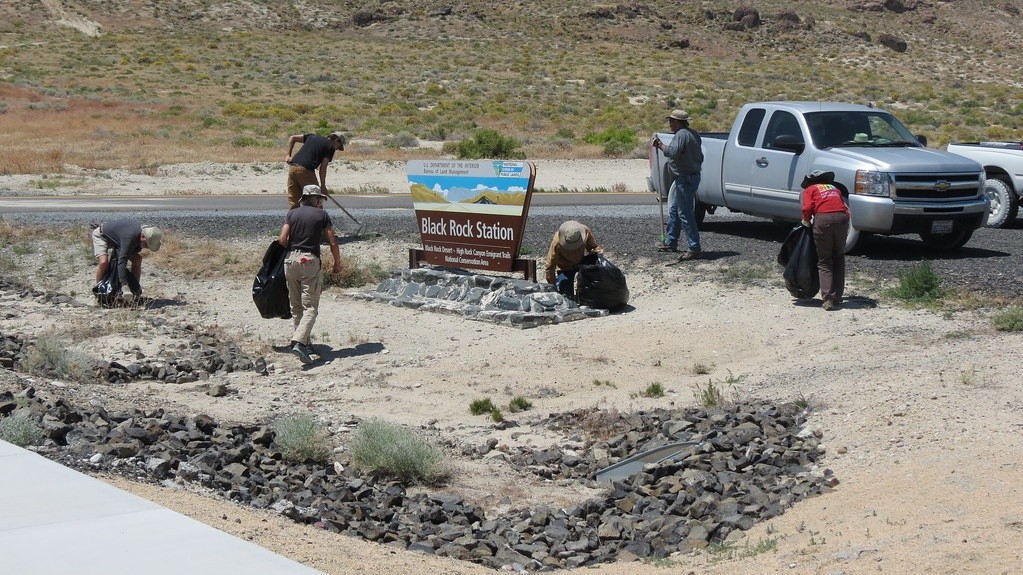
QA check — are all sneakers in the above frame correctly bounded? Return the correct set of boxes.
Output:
[292,343,313,364]
[822,299,840,310]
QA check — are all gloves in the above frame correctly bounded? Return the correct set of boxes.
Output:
[652,138,663,148]
[802,219,811,227]
[122,285,133,301]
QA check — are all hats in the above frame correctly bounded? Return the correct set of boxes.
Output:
[329,132,345,151]
[142,225,162,252]
[303,185,327,201]
[665,110,693,120]
[800,170,835,189]
[558,221,586,250]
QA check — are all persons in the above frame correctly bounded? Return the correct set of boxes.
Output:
[653,110,704,260]
[278,184,340,364]
[801,170,850,310]
[92,219,162,301]
[286,131,345,210]
[547,221,604,296]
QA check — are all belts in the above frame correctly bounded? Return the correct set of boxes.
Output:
[291,163,299,166]
[100,226,104,237]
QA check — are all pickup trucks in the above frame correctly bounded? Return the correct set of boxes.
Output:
[946,141,1023,229]
[646,100,990,254]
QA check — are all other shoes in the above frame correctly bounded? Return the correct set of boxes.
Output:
[657,243,677,252]
[678,252,703,260]
